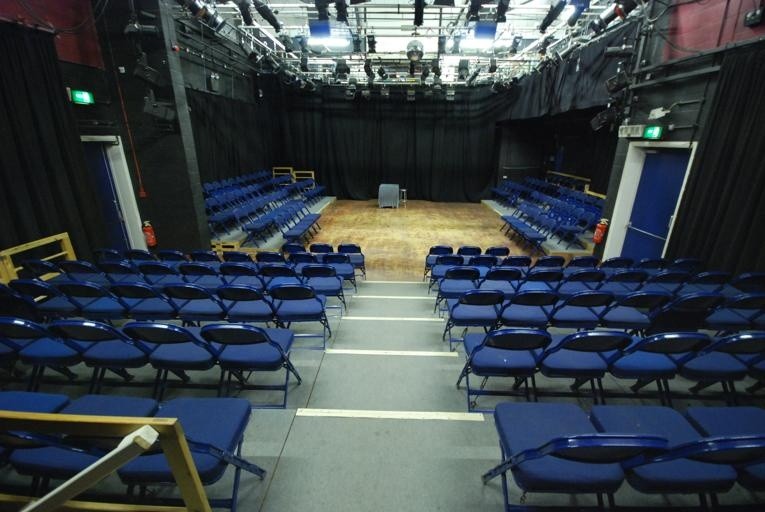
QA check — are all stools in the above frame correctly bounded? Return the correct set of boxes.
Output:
[400,189,408,209]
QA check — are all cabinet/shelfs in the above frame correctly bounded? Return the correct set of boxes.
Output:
[379,184,399,209]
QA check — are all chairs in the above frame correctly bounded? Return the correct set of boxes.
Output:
[434,267,480,314]
[274,216,304,242]
[495,290,560,330]
[634,257,670,271]
[263,169,270,177]
[589,290,673,330]
[200,323,302,409]
[516,215,548,251]
[457,328,552,412]
[517,269,564,292]
[733,352,765,394]
[248,173,254,181]
[535,198,561,229]
[95,259,165,282]
[255,251,288,270]
[485,246,510,264]
[478,268,522,294]
[423,245,453,281]
[287,208,314,240]
[233,187,247,204]
[685,271,731,297]
[491,176,524,206]
[520,173,586,194]
[179,262,237,289]
[310,243,334,262]
[213,181,222,190]
[669,257,703,270]
[557,269,607,294]
[550,208,586,239]
[92,249,130,265]
[202,201,231,235]
[281,243,306,257]
[289,252,320,275]
[528,196,552,226]
[302,263,347,316]
[0,338,39,378]
[442,290,506,352]
[227,187,242,206]
[242,175,247,183]
[338,243,366,280]
[258,263,308,289]
[257,171,263,178]
[212,192,237,216]
[467,255,498,276]
[297,201,321,230]
[531,331,634,405]
[243,204,279,238]
[457,245,482,264]
[507,204,538,241]
[732,272,762,298]
[557,211,595,250]
[498,256,532,276]
[221,179,228,188]
[124,249,158,266]
[250,200,284,232]
[428,255,464,295]
[641,270,692,295]
[0,389,71,472]
[254,183,266,196]
[219,188,238,208]
[558,186,570,201]
[516,191,540,218]
[115,398,265,512]
[482,403,670,512]
[269,284,332,350]
[60,260,127,283]
[201,186,205,194]
[248,185,260,199]
[510,207,542,246]
[546,205,576,239]
[236,176,242,184]
[604,270,649,297]
[541,290,616,332]
[593,199,606,224]
[232,207,267,248]
[21,260,96,282]
[46,318,190,393]
[667,331,765,405]
[228,178,235,187]
[163,282,237,327]
[8,279,100,319]
[599,257,635,271]
[569,189,581,205]
[686,406,765,494]
[278,212,310,244]
[706,293,765,336]
[52,280,147,325]
[190,250,222,272]
[206,197,239,230]
[269,194,295,218]
[218,284,282,327]
[241,186,254,202]
[273,191,300,216]
[0,316,98,390]
[139,260,201,283]
[322,253,357,294]
[222,251,256,268]
[220,262,273,291]
[281,189,305,210]
[524,218,557,257]
[205,184,214,194]
[642,291,725,333]
[543,201,568,234]
[589,404,731,512]
[530,256,566,270]
[122,322,226,399]
[500,203,528,236]
[575,192,588,207]
[295,177,325,207]
[293,204,318,234]
[265,194,294,222]
[258,197,290,229]
[156,250,190,272]
[9,395,159,495]
[270,175,298,197]
[106,281,190,326]
[598,332,712,408]
[582,196,597,213]
[566,256,600,268]
